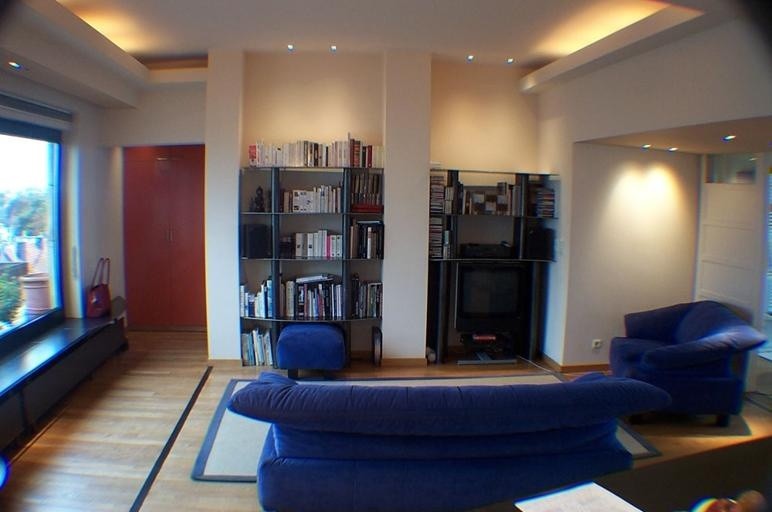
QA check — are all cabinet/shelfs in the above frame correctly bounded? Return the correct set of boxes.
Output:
[426,166,560,364]
[236,162,386,368]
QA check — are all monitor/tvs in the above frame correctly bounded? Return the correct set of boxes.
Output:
[453,261,531,332]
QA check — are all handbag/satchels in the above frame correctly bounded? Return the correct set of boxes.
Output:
[86,283,111,317]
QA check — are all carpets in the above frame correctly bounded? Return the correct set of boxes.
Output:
[191,370,662,482]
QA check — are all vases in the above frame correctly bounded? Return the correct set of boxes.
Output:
[18,272,51,314]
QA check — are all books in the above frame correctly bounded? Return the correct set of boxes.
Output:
[239,128,384,367]
[425,165,555,259]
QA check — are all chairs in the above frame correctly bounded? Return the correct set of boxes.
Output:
[608,299,768,433]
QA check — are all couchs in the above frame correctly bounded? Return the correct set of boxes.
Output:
[227,372,674,511]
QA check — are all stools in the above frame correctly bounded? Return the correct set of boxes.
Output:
[272,323,349,381]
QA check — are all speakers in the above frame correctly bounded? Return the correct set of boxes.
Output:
[528,228,554,259]
[240,222,267,258]
